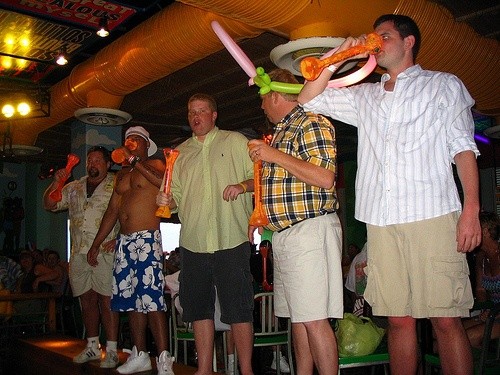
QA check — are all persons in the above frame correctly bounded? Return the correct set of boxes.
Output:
[249,239,291,374]
[42,145,120,369]
[156,94,255,374]
[0,249,69,334]
[297,14,481,375]
[87,126,176,375]
[247,68,344,375]
[433,210,500,375]
[162,248,240,375]
[345,241,368,314]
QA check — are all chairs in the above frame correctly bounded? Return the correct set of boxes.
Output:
[1,267,70,340]
[250,293,295,375]
[330,271,500,375]
[170,292,217,372]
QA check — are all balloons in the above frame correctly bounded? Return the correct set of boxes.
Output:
[211,20,376,95]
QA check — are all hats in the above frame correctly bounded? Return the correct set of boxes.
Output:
[125,126,157,157]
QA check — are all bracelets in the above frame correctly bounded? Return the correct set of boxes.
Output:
[240,183,247,193]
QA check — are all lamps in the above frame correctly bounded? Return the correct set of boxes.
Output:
[55,46,69,67]
[96,18,110,38]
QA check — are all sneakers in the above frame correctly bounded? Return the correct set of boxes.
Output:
[156,350,175,375]
[116,345,152,374]
[228,364,239,375]
[72,344,102,363]
[100,347,119,368]
[271,356,290,373]
